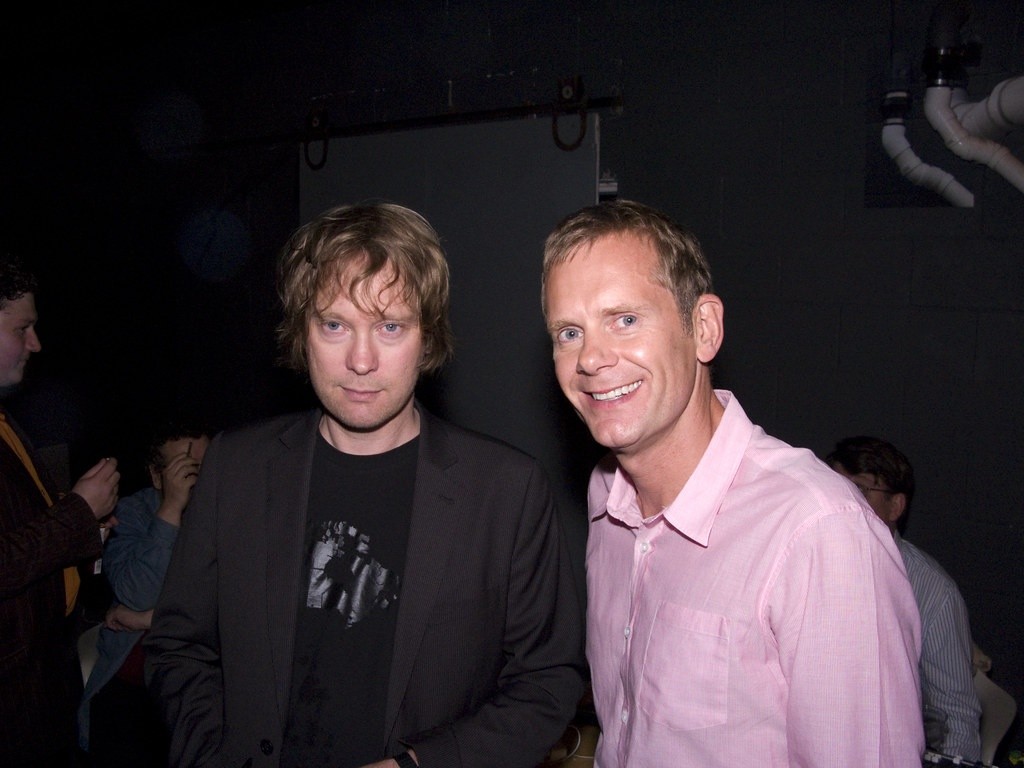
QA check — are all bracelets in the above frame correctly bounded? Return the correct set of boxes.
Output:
[393,751,417,768]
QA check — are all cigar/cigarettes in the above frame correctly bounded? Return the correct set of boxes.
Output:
[186,440,194,459]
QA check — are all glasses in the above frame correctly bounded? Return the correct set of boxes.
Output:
[856,483,893,499]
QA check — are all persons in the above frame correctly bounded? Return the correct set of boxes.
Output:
[133,197,587,768]
[0,251,982,768]
[540,199,926,768]
